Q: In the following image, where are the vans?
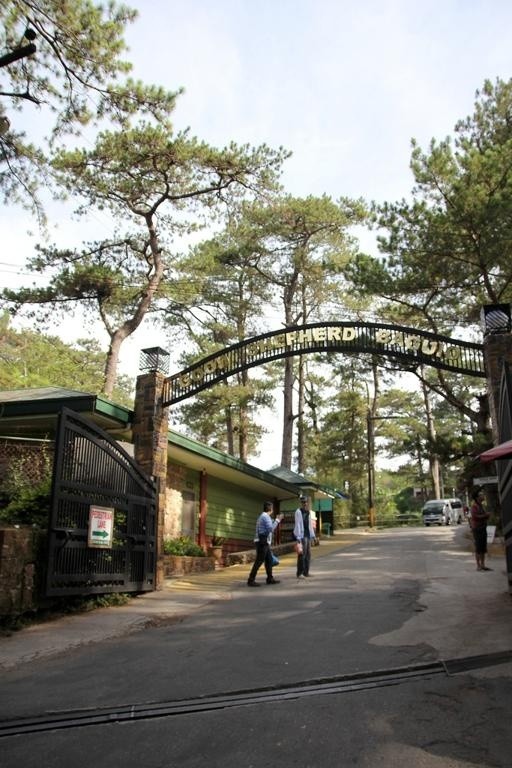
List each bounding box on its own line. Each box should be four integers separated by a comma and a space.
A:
422, 498, 464, 526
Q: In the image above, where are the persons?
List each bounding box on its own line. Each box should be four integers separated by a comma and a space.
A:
246, 502, 286, 587
292, 495, 318, 579
468, 489, 493, 572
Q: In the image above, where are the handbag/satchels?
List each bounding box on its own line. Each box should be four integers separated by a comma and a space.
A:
259, 534, 268, 543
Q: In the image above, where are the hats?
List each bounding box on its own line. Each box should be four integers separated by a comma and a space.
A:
298, 496, 307, 503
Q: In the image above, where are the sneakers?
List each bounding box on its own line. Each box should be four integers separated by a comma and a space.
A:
298, 574, 313, 580
266, 577, 281, 584
477, 567, 493, 572
247, 581, 261, 586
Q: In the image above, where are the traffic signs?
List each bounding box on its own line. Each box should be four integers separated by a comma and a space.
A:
87, 505, 115, 549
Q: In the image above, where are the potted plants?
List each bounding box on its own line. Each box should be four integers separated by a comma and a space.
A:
208, 534, 225, 559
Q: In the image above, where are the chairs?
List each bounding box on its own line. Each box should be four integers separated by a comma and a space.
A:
322, 523, 331, 536
